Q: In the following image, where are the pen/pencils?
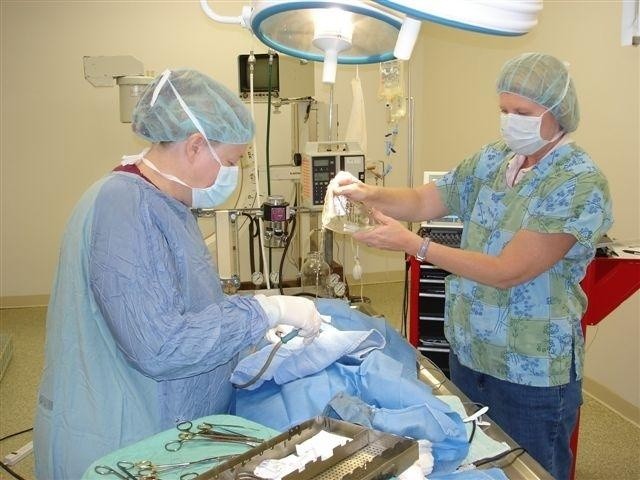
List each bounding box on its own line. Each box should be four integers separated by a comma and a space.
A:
623, 250, 640, 255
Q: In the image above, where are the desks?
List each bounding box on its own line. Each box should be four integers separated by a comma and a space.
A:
348, 298, 555, 480
82, 412, 283, 480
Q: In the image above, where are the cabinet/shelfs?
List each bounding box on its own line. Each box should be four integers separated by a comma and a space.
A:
405, 255, 454, 370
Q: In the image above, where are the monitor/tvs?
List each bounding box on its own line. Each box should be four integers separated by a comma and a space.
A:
237, 53, 315, 103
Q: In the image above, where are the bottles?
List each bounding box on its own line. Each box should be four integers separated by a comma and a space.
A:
296, 252, 330, 296
260, 194, 288, 249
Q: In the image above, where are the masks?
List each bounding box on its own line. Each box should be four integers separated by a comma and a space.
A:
182, 135, 240, 210
499, 99, 564, 158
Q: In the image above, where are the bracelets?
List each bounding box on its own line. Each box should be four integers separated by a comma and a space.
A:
413, 232, 433, 266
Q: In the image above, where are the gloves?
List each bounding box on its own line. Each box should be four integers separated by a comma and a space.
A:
254, 293, 321, 338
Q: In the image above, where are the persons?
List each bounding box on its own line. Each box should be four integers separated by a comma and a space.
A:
333, 52, 615, 478
30, 62, 333, 480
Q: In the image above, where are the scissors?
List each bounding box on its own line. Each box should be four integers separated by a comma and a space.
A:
95, 454, 264, 480
161, 419, 270, 455
